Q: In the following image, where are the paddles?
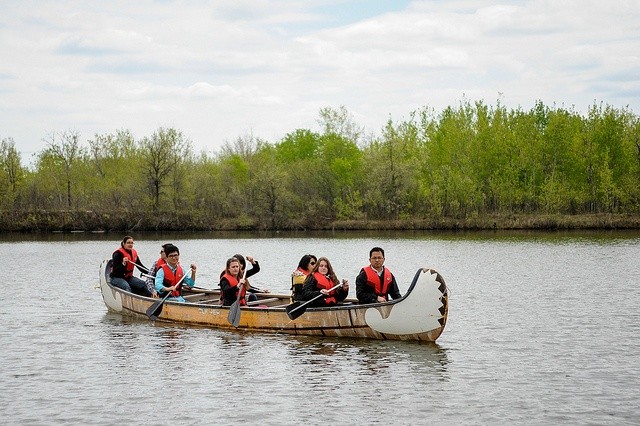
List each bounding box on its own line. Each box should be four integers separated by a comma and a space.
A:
285, 282, 343, 320
127, 259, 150, 272
140, 273, 220, 291
227, 259, 249, 328
145, 268, 192, 321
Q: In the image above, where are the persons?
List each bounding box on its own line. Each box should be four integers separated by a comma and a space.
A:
290, 255, 317, 304
355, 247, 402, 305
145, 244, 174, 299
109, 235, 152, 298
220, 256, 260, 308
219, 253, 271, 307
301, 257, 349, 307
155, 245, 196, 303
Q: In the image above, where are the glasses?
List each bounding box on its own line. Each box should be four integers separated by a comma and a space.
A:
371, 257, 384, 260
161, 250, 165, 253
309, 262, 315, 265
168, 254, 179, 257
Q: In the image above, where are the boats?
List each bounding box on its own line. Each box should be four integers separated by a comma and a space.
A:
99, 259, 449, 342
